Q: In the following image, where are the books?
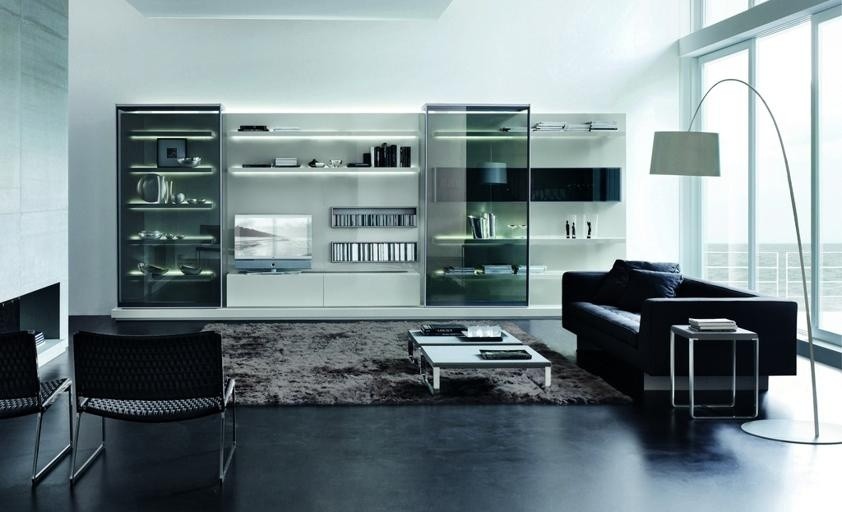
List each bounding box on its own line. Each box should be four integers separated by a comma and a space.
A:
237, 126, 300, 131
347, 142, 411, 167
420, 323, 469, 336
468, 212, 496, 239
688, 318, 738, 332
500, 120, 619, 133
479, 349, 532, 360
443, 263, 547, 276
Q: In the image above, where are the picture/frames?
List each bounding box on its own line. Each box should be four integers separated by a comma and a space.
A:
156, 137, 188, 168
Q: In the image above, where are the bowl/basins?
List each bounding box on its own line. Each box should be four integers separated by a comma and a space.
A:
176, 157, 201, 167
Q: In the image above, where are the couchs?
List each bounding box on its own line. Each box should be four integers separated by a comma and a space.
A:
559, 270, 798, 394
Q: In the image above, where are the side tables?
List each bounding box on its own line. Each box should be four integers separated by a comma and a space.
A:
668, 323, 760, 423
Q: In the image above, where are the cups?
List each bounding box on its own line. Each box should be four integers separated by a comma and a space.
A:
157, 176, 176, 205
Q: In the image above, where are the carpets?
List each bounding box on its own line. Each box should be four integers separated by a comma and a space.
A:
201, 320, 634, 408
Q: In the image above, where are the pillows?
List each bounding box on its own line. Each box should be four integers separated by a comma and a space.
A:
599, 258, 684, 314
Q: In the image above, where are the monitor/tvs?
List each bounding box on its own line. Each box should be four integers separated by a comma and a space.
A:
233, 212, 313, 273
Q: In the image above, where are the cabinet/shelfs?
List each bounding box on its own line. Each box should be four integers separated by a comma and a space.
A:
109, 102, 626, 323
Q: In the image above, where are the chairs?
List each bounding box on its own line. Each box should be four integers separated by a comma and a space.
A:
0, 328, 238, 489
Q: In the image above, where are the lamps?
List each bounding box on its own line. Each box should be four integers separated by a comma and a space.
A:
648, 76, 842, 447
